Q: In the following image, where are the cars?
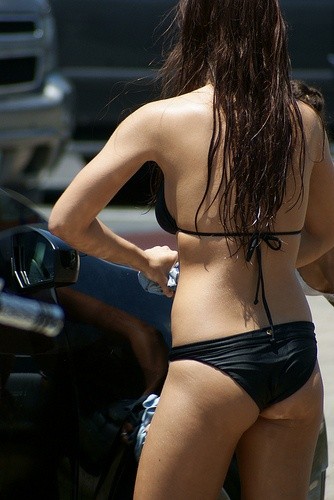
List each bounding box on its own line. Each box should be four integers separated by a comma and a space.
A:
1, 1, 333, 208
2, 188, 252, 499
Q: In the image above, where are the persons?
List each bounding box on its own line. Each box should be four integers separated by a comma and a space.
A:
47, 0, 332, 500
285, 80, 334, 306
